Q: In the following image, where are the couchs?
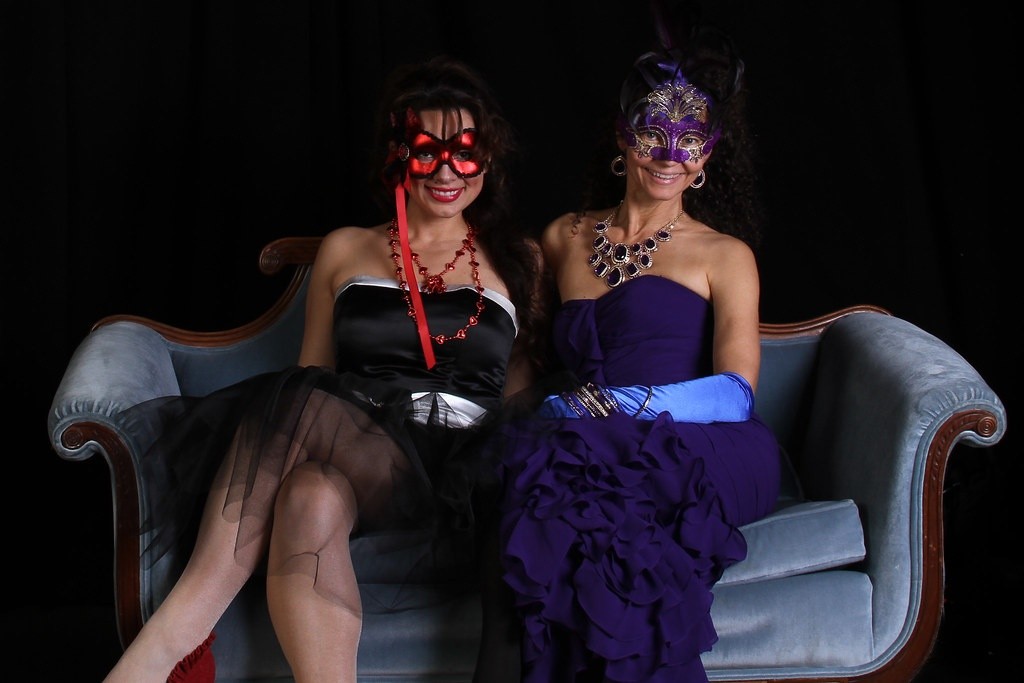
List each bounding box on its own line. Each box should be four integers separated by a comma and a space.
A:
46, 232, 1011, 683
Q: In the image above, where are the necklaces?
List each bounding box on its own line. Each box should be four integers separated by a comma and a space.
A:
386, 207, 486, 345
588, 198, 686, 292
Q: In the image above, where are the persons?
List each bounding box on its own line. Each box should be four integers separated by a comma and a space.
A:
91, 54, 540, 683
498, 51, 769, 682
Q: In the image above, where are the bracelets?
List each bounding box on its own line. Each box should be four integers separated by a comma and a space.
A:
559, 376, 654, 418
